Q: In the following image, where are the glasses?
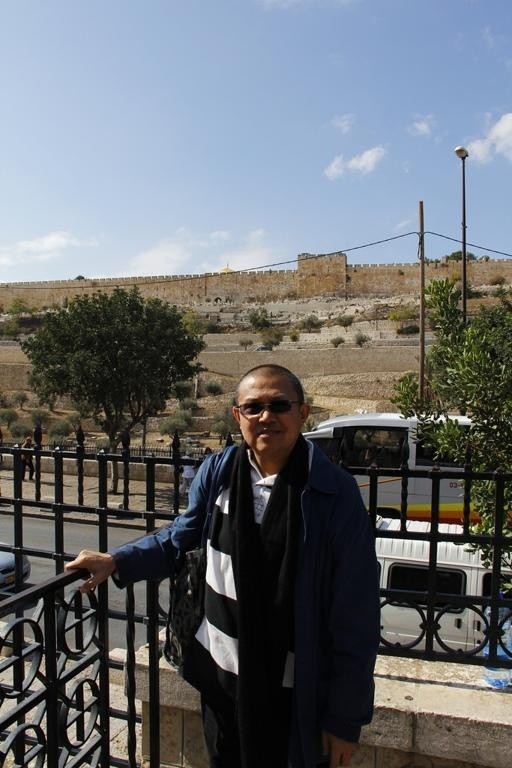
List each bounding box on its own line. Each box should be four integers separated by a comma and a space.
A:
236, 399, 301, 415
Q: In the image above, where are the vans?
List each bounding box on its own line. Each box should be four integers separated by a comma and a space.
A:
363, 515, 512, 656
301, 412, 512, 526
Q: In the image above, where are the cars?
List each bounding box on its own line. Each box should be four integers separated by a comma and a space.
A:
0, 540, 32, 592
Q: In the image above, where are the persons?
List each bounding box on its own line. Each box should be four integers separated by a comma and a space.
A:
64, 363, 381, 768
179, 448, 199, 496
202, 447, 212, 461
22, 436, 38, 482
187, 435, 192, 444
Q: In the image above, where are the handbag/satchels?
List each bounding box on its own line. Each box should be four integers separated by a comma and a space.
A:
163, 547, 207, 670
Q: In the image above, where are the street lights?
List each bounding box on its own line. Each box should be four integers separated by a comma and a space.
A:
449, 141, 471, 330
